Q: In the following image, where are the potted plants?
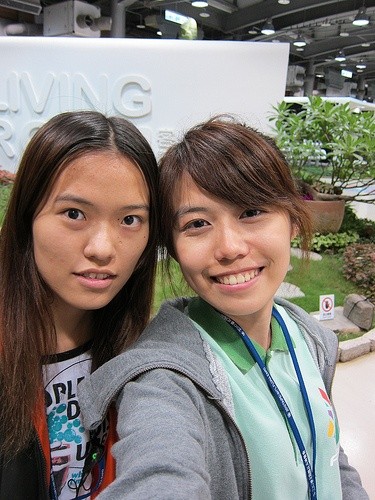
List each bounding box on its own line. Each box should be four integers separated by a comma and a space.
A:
269, 94, 375, 234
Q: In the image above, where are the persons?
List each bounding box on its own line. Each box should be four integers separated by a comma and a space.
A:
78, 115, 368, 500
0, 110, 159, 500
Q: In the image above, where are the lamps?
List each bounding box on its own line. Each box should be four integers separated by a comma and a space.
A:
261, 15, 275, 34
335, 48, 346, 62
352, 0, 371, 26
